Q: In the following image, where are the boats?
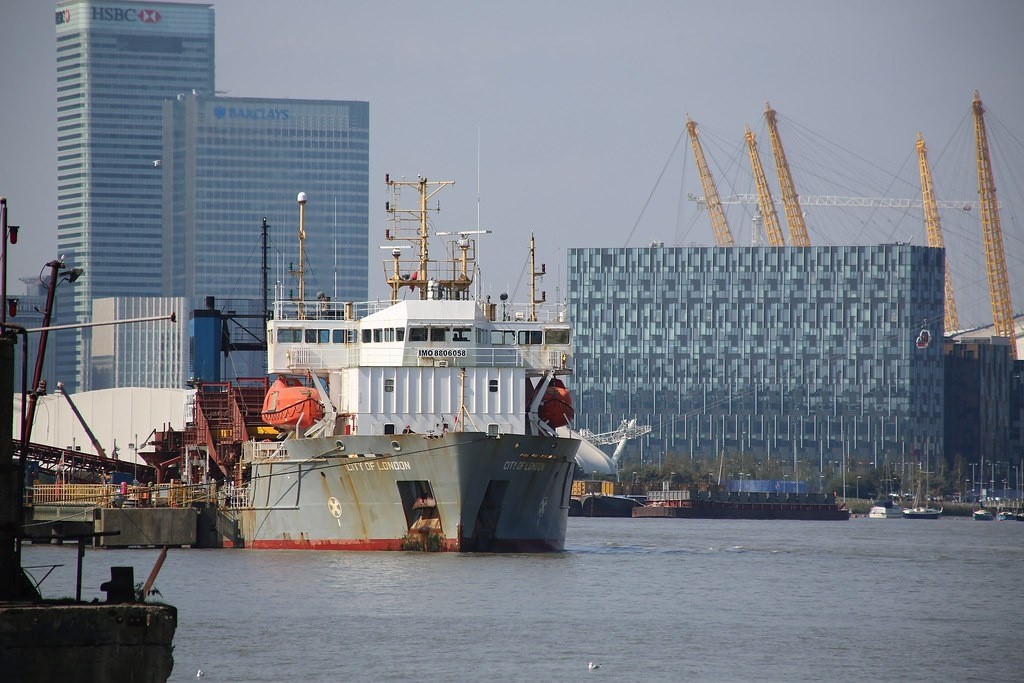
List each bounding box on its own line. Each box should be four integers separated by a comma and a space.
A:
996, 511, 1015, 521
1, 174, 853, 682
870, 506, 904, 520
1014, 513, 1024, 520
973, 510, 994, 520
903, 505, 945, 519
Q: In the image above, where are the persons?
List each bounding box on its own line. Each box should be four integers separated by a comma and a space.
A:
403, 425, 415, 434
